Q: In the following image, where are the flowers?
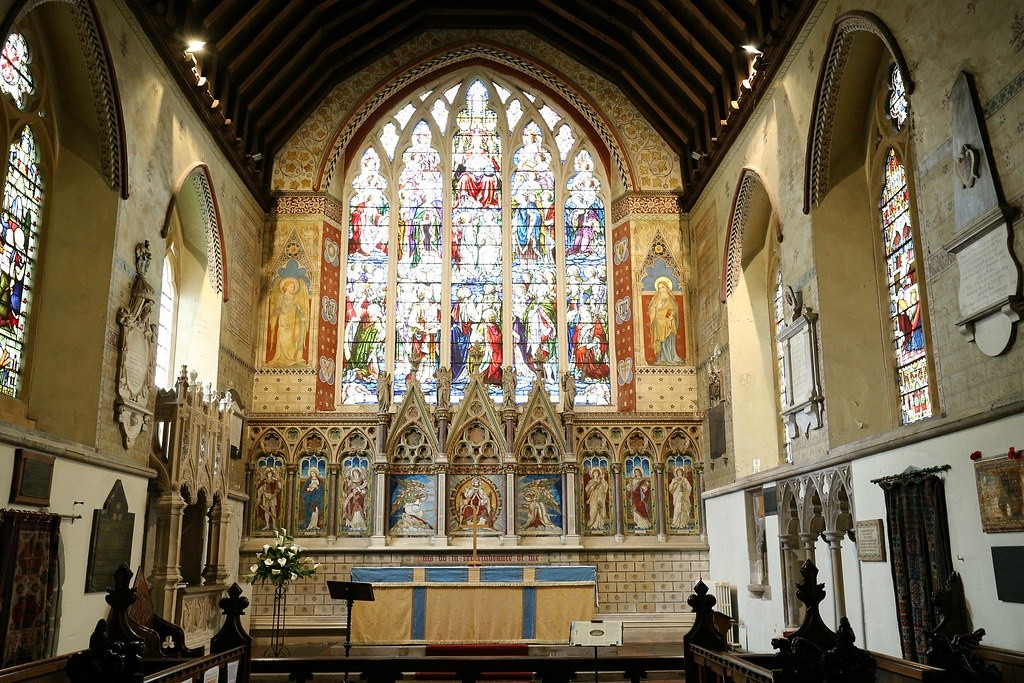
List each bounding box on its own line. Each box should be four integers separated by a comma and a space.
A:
242, 527, 320, 586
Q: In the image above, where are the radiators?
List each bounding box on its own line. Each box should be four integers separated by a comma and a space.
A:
694, 579, 736, 651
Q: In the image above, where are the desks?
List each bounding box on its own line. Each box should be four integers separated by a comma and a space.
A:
348, 565, 598, 645
